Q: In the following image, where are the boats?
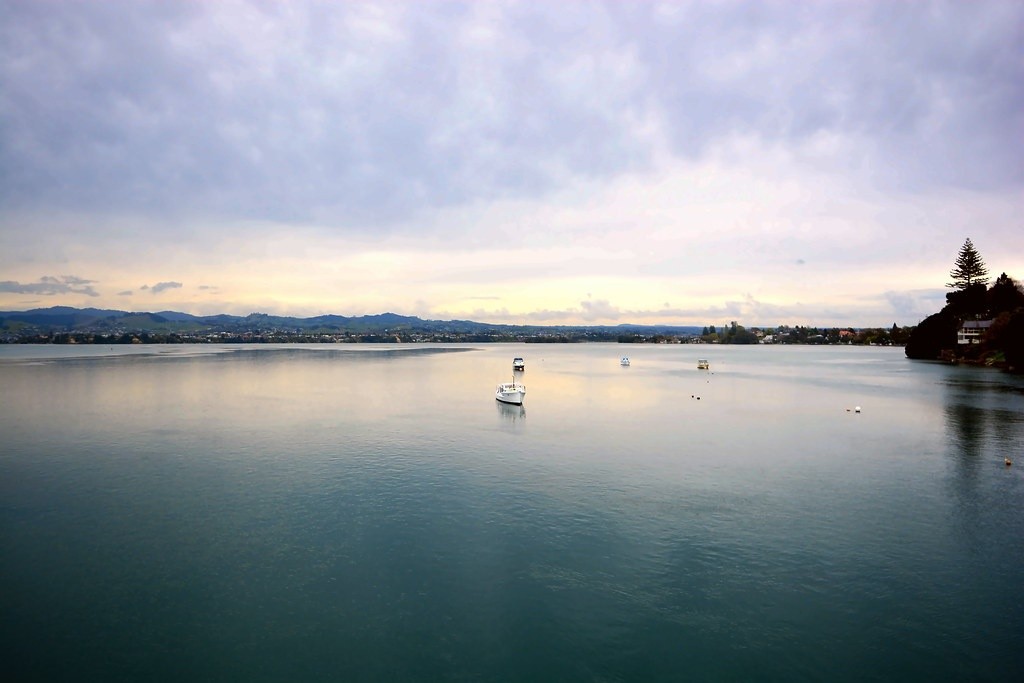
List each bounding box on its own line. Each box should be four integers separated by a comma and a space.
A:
495, 375, 525, 403
621, 357, 630, 365
512, 357, 524, 369
697, 359, 709, 369
